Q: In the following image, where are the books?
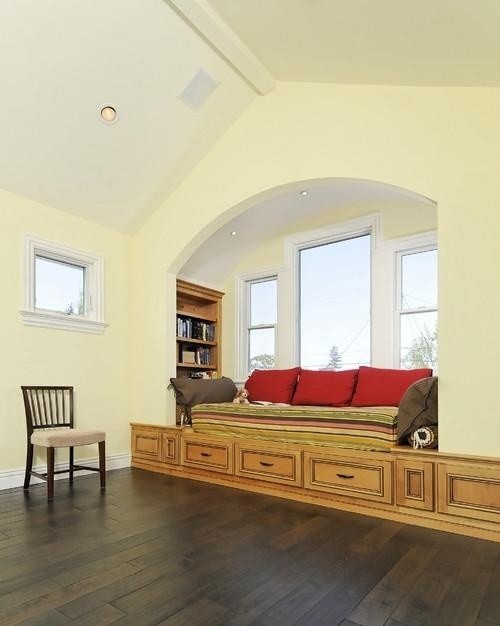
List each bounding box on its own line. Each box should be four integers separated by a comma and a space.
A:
176, 316, 217, 380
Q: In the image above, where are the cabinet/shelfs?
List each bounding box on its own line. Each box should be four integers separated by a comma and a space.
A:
130, 424, 500, 548
177, 281, 225, 379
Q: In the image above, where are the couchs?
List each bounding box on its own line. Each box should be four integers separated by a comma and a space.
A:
169, 365, 436, 451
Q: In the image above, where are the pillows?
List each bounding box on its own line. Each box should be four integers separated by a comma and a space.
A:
170, 367, 438, 439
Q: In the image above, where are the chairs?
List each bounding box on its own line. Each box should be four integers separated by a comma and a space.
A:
20, 387, 106, 500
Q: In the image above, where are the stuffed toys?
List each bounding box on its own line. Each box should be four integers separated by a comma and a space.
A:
232, 389, 251, 404
